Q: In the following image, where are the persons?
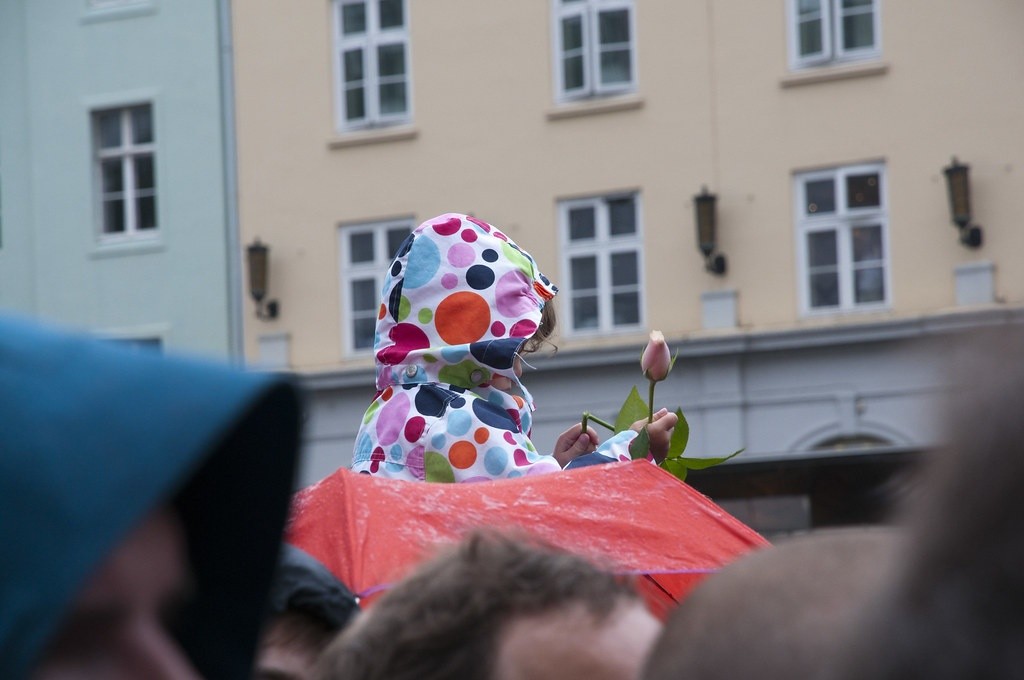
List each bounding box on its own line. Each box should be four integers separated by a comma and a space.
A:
246, 536, 365, 680
344, 212, 682, 479
0, 302, 314, 680
303, 525, 668, 680
866, 339, 1023, 678
641, 518, 927, 680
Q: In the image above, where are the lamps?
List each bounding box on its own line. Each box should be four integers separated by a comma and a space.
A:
247, 238, 278, 320
943, 157, 981, 246
693, 187, 725, 275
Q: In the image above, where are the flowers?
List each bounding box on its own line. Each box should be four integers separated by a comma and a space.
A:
581, 329, 745, 484
